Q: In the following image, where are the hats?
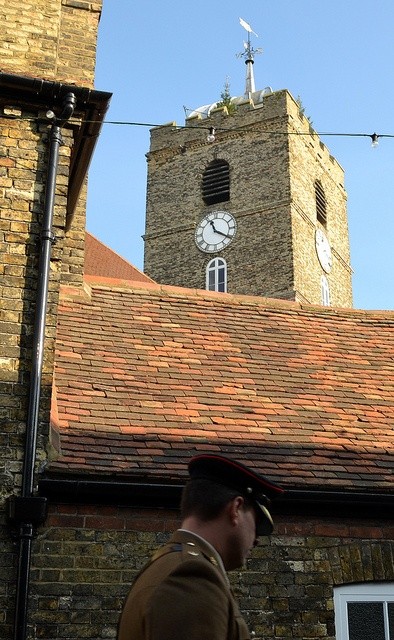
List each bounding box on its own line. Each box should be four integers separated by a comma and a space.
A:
188, 454, 284, 535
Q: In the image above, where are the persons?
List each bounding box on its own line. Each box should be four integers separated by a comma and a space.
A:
116, 454, 284, 640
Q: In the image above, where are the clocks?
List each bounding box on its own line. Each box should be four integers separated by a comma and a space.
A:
315, 229, 333, 274
193, 209, 238, 255
